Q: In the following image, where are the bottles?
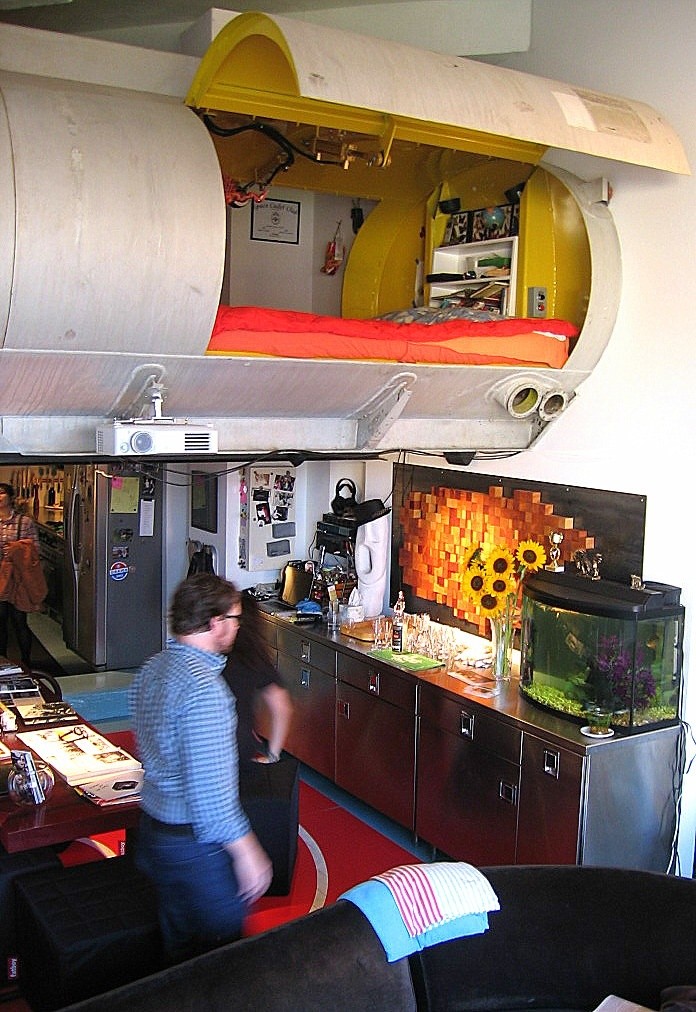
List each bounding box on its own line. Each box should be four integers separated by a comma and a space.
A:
391, 598, 408, 654
8, 760, 54, 808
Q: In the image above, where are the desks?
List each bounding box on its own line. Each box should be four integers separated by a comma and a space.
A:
0, 652, 146, 865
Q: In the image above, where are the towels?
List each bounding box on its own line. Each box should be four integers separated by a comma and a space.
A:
336, 861, 502, 964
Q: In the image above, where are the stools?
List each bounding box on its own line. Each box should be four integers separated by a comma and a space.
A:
11, 854, 206, 1012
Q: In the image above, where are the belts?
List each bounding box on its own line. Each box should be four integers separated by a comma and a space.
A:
138, 811, 198, 836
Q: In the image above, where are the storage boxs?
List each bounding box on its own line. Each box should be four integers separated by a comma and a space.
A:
519, 584, 686, 733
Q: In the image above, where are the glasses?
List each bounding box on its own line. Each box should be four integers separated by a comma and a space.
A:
0, 490, 7, 494
224, 613, 245, 624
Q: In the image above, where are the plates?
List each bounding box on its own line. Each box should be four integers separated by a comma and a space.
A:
580, 726, 614, 738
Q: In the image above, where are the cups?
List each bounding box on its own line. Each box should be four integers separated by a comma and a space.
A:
345, 617, 355, 629
371, 612, 455, 672
327, 601, 342, 631
587, 706, 613, 735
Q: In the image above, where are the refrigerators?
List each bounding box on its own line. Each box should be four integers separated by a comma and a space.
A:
61, 463, 161, 669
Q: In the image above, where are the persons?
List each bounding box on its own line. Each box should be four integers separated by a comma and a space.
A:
221, 591, 302, 898
128, 571, 274, 966
0, 481, 48, 670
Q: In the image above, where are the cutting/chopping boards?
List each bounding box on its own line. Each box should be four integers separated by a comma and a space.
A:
339, 616, 393, 642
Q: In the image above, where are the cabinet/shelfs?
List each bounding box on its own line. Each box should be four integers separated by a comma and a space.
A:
247, 616, 585, 872
426, 237, 518, 316
